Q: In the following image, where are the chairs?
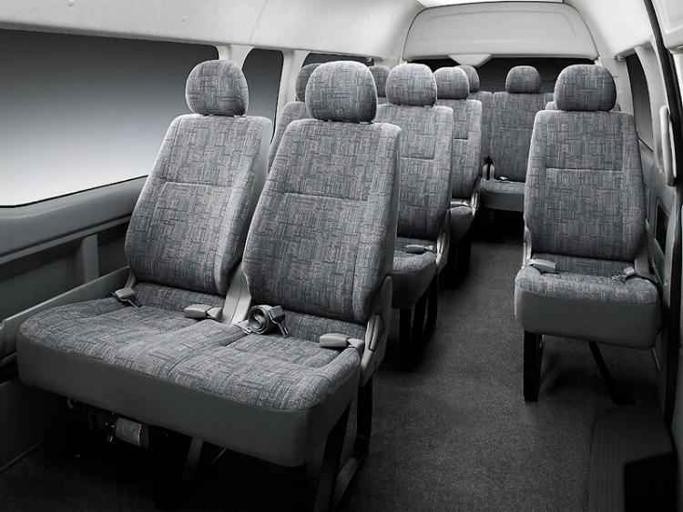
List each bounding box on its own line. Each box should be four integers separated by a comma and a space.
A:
510, 63, 662, 407
266, 59, 551, 374
14, 59, 405, 507
